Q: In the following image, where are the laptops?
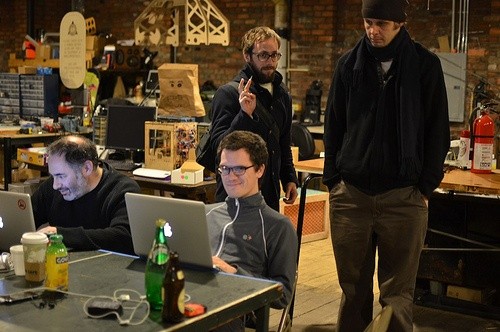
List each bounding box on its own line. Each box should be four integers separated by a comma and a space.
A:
125, 193, 220, 272
0, 191, 36, 252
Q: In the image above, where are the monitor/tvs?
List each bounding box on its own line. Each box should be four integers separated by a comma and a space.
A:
145, 70, 160, 93
105, 105, 156, 163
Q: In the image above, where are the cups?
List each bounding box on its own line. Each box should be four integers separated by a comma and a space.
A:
134, 88, 142, 97
21, 232, 49, 281
10, 245, 25, 275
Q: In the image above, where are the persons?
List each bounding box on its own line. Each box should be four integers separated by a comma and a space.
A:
28, 135, 143, 256
196, 28, 299, 214
202, 132, 297, 332
321, 0, 451, 332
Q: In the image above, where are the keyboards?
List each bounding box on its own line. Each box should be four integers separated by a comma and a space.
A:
104, 160, 135, 171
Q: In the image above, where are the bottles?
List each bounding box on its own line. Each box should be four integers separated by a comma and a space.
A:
145, 226, 170, 310
492, 154, 496, 170
46, 235, 68, 291
451, 141, 460, 159
458, 130, 470, 167
145, 96, 156, 107
321, 112, 324, 123
164, 251, 186, 326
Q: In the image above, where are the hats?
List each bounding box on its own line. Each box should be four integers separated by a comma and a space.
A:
361, 0, 406, 22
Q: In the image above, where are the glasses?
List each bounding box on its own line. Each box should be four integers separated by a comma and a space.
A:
216, 165, 255, 176
250, 51, 281, 62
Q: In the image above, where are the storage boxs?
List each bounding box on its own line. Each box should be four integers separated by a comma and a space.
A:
16, 148, 48, 166
0, 73, 60, 121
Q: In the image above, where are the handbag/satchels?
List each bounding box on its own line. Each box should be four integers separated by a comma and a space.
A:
195, 81, 252, 174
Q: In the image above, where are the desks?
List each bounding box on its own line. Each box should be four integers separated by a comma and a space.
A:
0, 130, 220, 205
284, 157, 500, 327
0, 248, 285, 332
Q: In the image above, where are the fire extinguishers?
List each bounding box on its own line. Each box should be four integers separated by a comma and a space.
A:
470, 102, 500, 174
